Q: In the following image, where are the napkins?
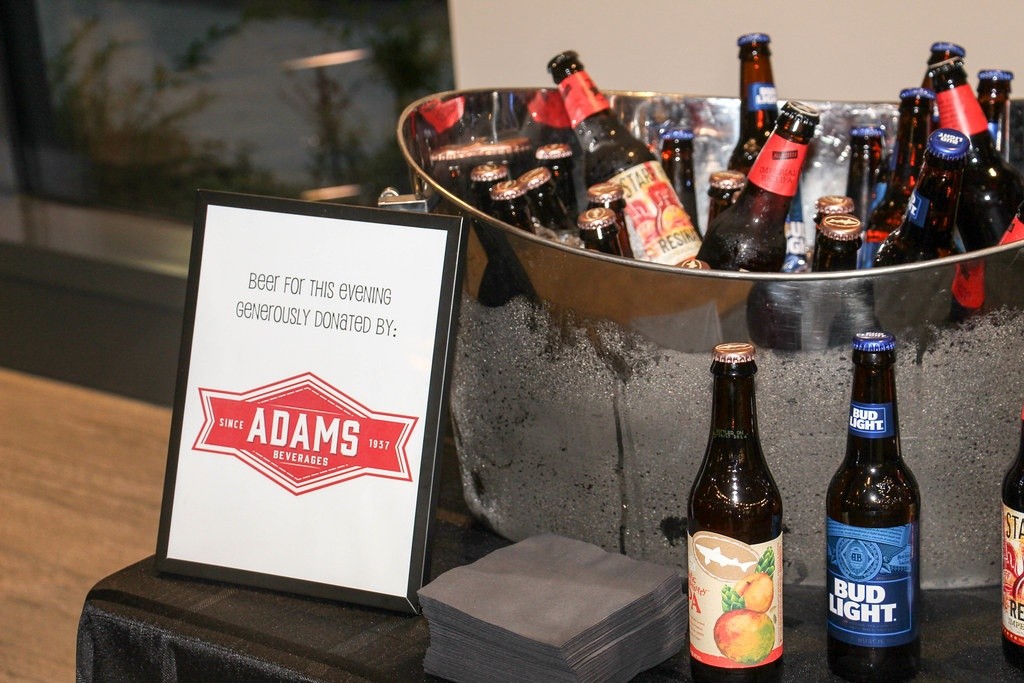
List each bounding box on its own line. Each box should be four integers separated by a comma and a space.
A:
416, 535, 692, 683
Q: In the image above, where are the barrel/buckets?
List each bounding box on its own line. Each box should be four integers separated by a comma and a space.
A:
394, 84, 1024, 591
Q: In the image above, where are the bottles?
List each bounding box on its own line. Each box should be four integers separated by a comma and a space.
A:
999, 403, 1024, 662
824, 330, 923, 683
467, 143, 636, 267
811, 121, 884, 274
693, 28, 821, 274
685, 340, 785, 683
546, 46, 702, 267
864, 39, 1024, 269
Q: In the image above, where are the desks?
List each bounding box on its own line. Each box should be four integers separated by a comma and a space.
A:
76, 511, 1024, 683
